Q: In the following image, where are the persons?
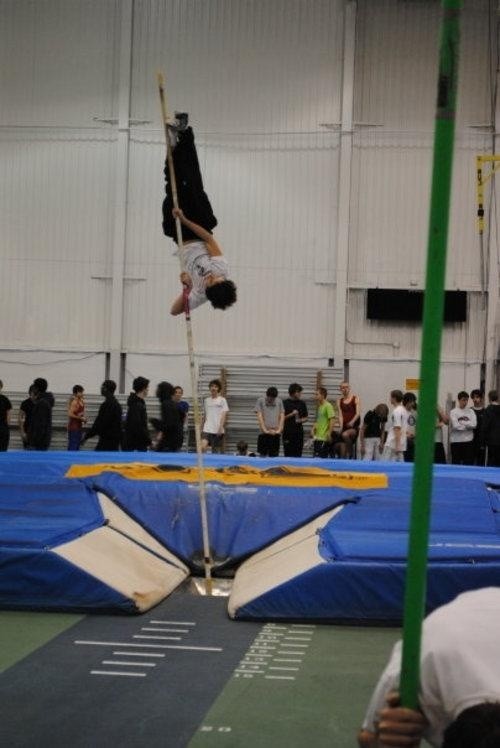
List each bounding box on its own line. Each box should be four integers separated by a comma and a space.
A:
68, 385, 86, 451
381, 390, 406, 462
359, 586, 500, 748
338, 382, 360, 458
436, 404, 449, 464
449, 392, 476, 466
360, 404, 389, 463
163, 111, 237, 315
402, 392, 417, 462
149, 382, 183, 452
81, 380, 122, 451
19, 378, 54, 446
234, 441, 255, 457
255, 387, 284, 457
0, 380, 11, 451
470, 390, 487, 466
125, 377, 157, 451
26, 385, 52, 450
203, 380, 229, 453
311, 388, 335, 458
485, 391, 500, 467
174, 387, 189, 431
282, 384, 307, 457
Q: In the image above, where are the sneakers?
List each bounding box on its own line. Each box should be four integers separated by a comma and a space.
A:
176, 109, 187, 130
164, 121, 176, 139
